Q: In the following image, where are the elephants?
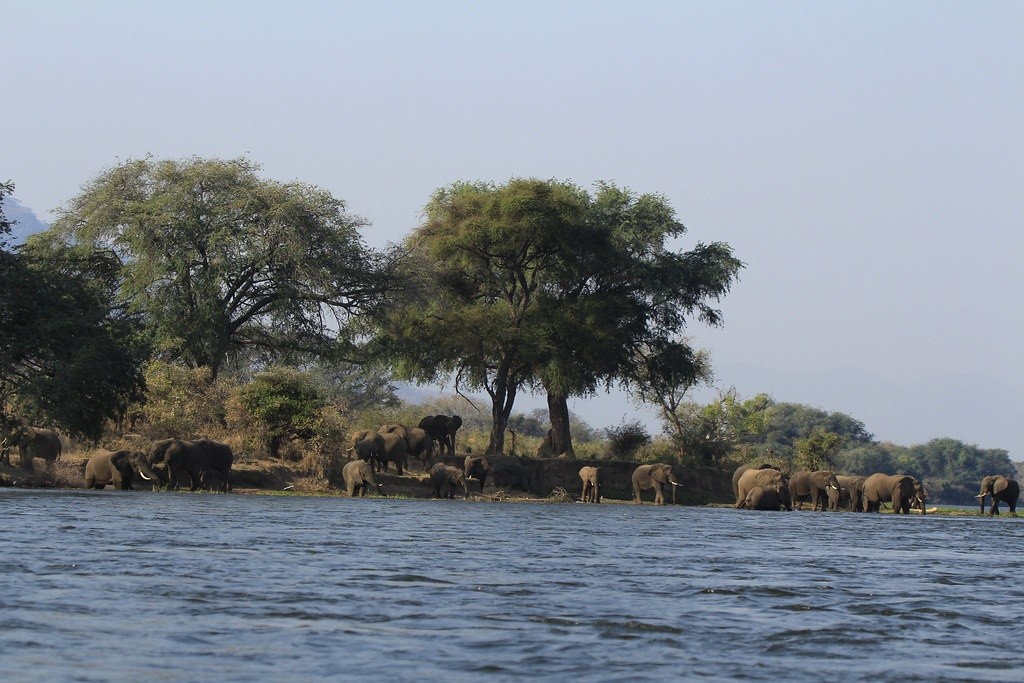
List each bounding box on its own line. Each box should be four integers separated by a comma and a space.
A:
0, 414, 1020, 518
